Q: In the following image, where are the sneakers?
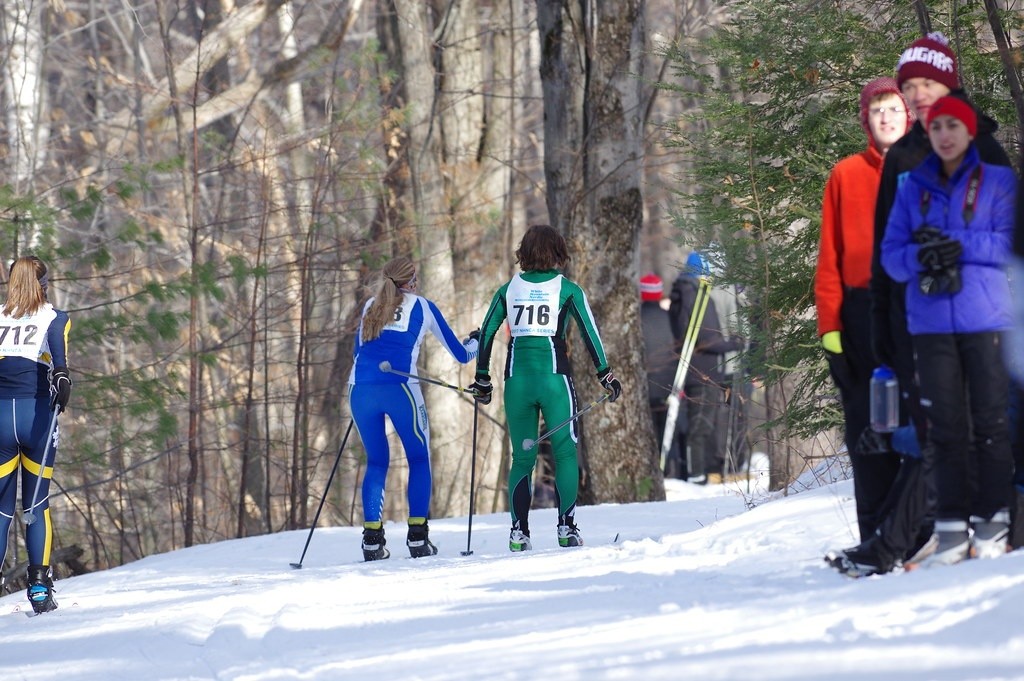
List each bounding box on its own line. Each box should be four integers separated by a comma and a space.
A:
556, 523, 585, 547
509, 528, 533, 552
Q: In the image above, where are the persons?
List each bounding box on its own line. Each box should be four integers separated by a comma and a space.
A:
470, 223, 623, 554
637, 252, 750, 486
868, 33, 1021, 526
813, 78, 933, 575
878, 89, 1024, 535
0, 256, 72, 613
346, 255, 479, 562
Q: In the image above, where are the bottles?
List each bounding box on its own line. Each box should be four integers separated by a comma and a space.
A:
868, 360, 899, 433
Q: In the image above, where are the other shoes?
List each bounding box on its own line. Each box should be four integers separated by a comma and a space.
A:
688, 473, 723, 484
822, 518, 938, 580
966, 505, 1012, 560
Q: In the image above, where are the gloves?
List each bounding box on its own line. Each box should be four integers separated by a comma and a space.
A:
871, 325, 891, 369
731, 331, 744, 349
823, 330, 849, 371
50, 368, 72, 413
469, 328, 480, 340
913, 226, 942, 243
916, 239, 964, 272
597, 366, 622, 402
472, 374, 493, 405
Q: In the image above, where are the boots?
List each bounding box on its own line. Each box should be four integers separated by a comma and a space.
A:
26, 565, 58, 614
361, 520, 391, 562
406, 518, 438, 558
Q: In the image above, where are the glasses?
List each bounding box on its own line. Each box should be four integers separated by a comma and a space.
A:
870, 104, 905, 116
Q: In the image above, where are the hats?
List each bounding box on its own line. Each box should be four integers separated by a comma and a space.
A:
897, 31, 958, 90
684, 253, 711, 278
861, 76, 913, 145
924, 88, 977, 137
639, 274, 664, 302
